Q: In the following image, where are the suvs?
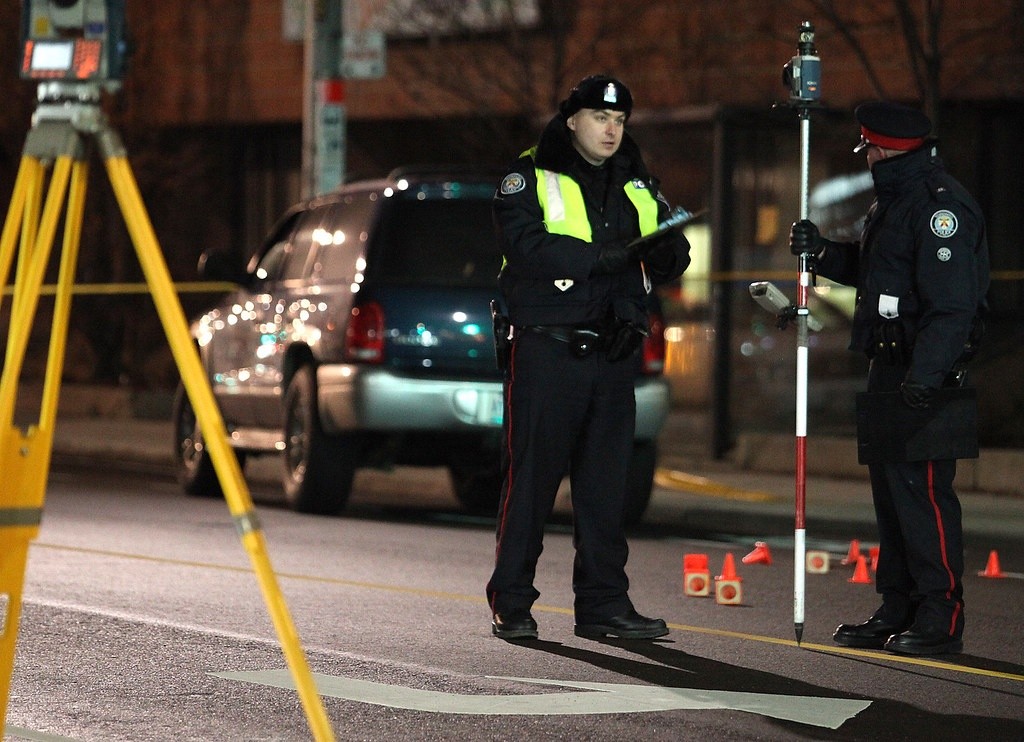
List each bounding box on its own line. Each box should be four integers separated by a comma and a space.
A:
171, 175, 670, 538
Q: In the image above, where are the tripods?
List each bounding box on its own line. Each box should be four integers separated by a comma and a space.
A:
0, 78, 339, 742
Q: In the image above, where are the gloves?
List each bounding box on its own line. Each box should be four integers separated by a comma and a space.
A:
594, 237, 646, 275
651, 225, 691, 275
788, 220, 822, 260
900, 379, 935, 410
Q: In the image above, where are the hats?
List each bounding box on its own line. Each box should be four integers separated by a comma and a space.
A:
568, 74, 631, 122
853, 102, 933, 155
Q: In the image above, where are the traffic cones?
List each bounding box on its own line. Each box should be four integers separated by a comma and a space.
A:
805, 550, 830, 575
682, 553, 711, 597
715, 580, 743, 607
840, 539, 861, 565
847, 556, 871, 584
869, 547, 881, 573
714, 550, 742, 583
744, 542, 772, 566
978, 550, 1009, 580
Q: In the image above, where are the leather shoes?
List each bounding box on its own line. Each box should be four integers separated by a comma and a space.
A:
574, 591, 669, 639
832, 604, 910, 649
491, 606, 538, 640
883, 624, 964, 655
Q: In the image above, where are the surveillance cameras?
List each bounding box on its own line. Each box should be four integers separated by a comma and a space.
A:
747, 282, 792, 315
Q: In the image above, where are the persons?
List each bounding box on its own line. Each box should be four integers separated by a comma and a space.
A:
486, 73, 692, 639
788, 96, 988, 657
